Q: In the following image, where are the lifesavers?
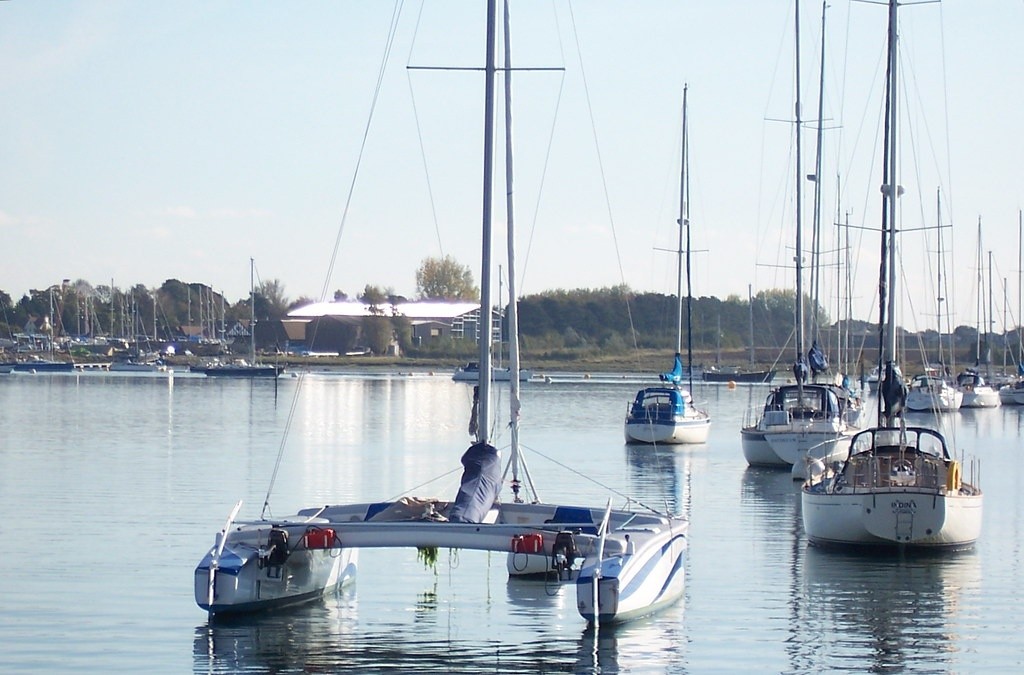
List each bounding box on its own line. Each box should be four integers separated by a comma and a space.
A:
946, 461, 962, 492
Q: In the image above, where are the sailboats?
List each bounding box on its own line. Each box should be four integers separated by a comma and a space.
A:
451, 264, 534, 382
739, 0, 1023, 553
194, 0, 691, 628
188, 257, 289, 378
701, 283, 779, 383
624, 82, 713, 446
0, 275, 225, 379
